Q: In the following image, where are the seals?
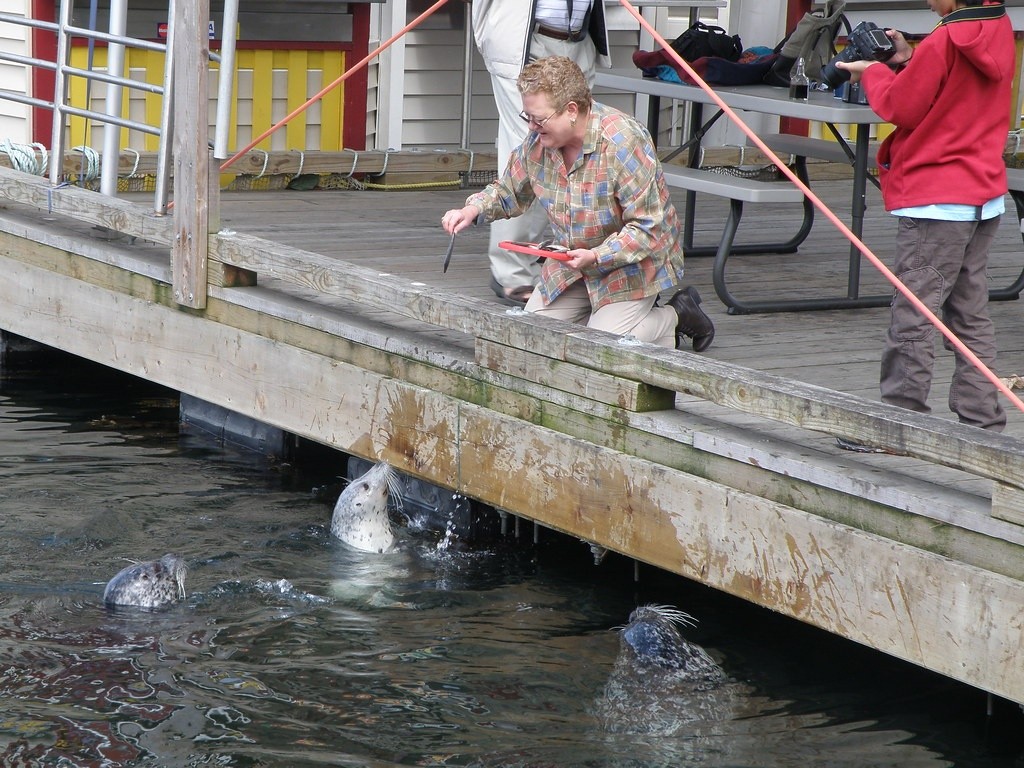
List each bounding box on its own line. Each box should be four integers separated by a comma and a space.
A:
104, 553, 189, 610
593, 605, 743, 766
328, 459, 411, 606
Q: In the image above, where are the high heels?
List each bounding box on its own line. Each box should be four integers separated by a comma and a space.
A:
665, 287, 714, 351
651, 293, 682, 350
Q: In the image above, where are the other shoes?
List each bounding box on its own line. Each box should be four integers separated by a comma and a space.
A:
836, 436, 895, 452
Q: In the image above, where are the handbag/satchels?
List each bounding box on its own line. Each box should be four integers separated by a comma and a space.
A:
634, 1, 854, 91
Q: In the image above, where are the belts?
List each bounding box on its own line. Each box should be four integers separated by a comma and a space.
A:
531, 22, 589, 41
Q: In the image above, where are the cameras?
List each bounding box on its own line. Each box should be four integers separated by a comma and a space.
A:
820, 21, 897, 90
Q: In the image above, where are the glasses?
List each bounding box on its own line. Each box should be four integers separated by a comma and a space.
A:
519, 99, 577, 131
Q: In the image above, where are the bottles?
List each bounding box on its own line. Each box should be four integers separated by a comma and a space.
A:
788, 57, 809, 103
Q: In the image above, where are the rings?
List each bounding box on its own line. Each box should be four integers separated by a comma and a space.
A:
441, 217, 444, 222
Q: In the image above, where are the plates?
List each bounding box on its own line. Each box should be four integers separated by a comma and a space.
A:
498, 240, 575, 261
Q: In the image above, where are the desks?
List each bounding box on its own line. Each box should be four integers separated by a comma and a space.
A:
588, 63, 1024, 311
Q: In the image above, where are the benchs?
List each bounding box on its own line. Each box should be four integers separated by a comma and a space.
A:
656, 131, 1023, 311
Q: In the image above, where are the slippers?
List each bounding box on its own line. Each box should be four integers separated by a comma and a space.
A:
490, 279, 536, 307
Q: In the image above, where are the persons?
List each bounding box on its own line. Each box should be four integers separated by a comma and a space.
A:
835, 0, 1016, 452
442, 56, 715, 353
471, 0, 612, 307
